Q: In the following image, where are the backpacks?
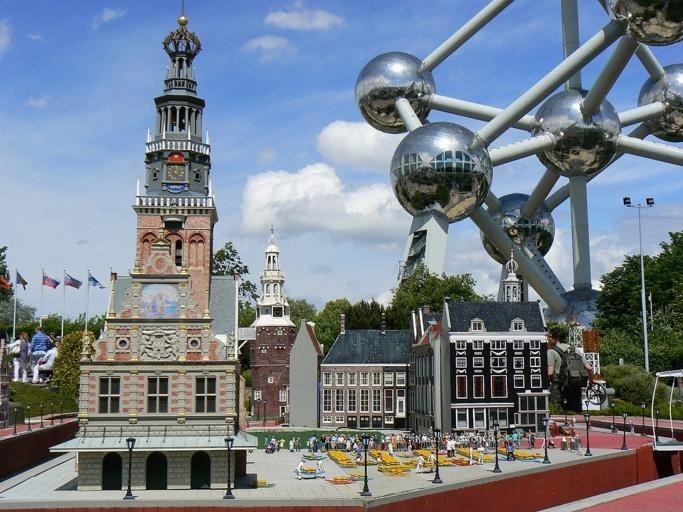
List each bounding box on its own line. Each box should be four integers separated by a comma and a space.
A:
551, 345, 589, 389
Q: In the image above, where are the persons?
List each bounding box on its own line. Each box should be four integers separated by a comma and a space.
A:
263, 417, 582, 480
546, 326, 593, 413
5, 326, 62, 384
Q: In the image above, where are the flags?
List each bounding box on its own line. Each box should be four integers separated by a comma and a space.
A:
0, 274, 10, 289
41, 270, 60, 290
63, 271, 82, 289
87, 271, 107, 290
16, 271, 28, 291
